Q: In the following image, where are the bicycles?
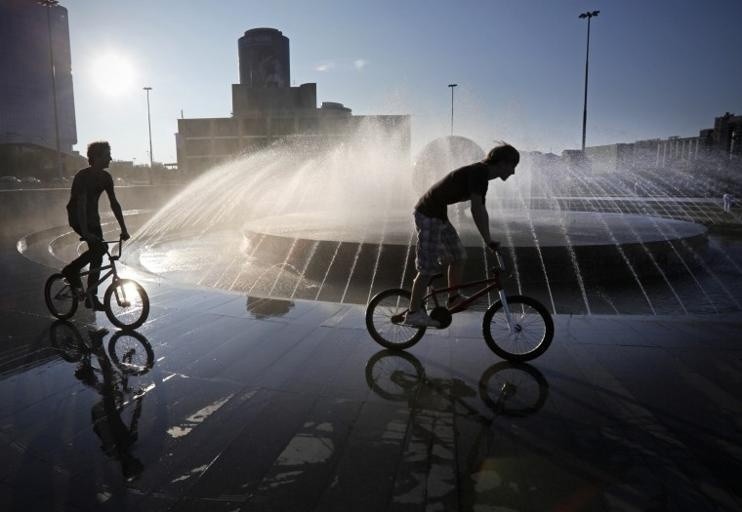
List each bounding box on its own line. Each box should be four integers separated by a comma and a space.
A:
44, 233, 149, 330
366, 245, 554, 362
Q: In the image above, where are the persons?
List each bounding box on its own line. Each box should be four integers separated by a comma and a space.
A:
402, 143, 520, 327
60, 139, 131, 312
722, 188, 735, 214
72, 326, 147, 486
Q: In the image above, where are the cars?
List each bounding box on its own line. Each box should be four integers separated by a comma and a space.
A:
0, 176, 75, 188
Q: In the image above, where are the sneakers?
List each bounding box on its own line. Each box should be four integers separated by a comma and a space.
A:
446, 294, 470, 307
85, 296, 104, 310
61, 265, 83, 287
405, 308, 440, 327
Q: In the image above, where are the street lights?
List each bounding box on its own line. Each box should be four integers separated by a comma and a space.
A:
578, 10, 599, 175
143, 87, 153, 185
449, 84, 458, 137
